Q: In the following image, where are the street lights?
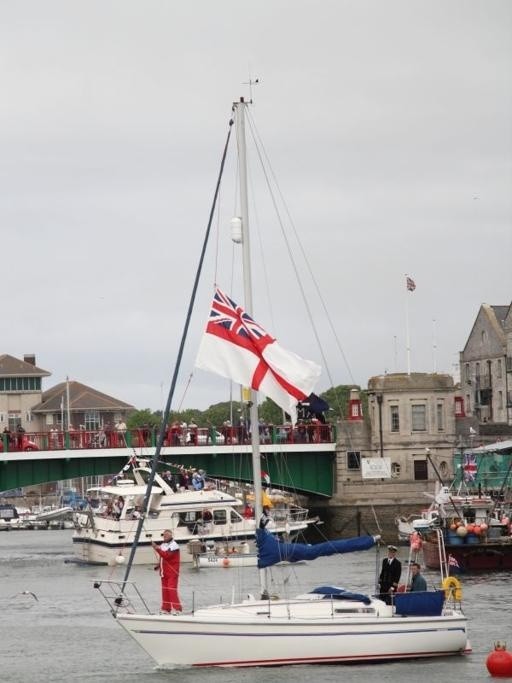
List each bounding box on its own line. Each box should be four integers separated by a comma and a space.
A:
374, 391, 386, 456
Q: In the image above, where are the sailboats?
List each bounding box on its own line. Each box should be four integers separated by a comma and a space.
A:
90, 73, 476, 674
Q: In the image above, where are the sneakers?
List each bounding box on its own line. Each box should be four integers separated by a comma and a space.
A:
157, 610, 172, 616
172, 610, 184, 616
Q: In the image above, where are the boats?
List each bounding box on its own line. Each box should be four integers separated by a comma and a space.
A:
413, 428, 511, 577
66, 450, 326, 567
0, 496, 79, 531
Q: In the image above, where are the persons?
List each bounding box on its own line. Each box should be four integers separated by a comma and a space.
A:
258, 418, 266, 435
50, 425, 59, 448
96, 419, 126, 447
410, 563, 427, 591
284, 413, 318, 443
3, 425, 25, 449
170, 419, 197, 444
375, 544, 401, 605
98, 452, 272, 523
224, 415, 246, 445
152, 531, 186, 615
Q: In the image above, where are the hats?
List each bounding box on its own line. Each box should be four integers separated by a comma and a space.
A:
387, 544, 398, 553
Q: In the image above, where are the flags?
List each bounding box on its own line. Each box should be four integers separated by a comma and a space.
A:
197, 289, 318, 417
460, 453, 478, 484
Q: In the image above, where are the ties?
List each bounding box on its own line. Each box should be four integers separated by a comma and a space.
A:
388, 560, 391, 565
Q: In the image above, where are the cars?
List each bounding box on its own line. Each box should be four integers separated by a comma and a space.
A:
184, 423, 289, 445
1, 436, 38, 451
